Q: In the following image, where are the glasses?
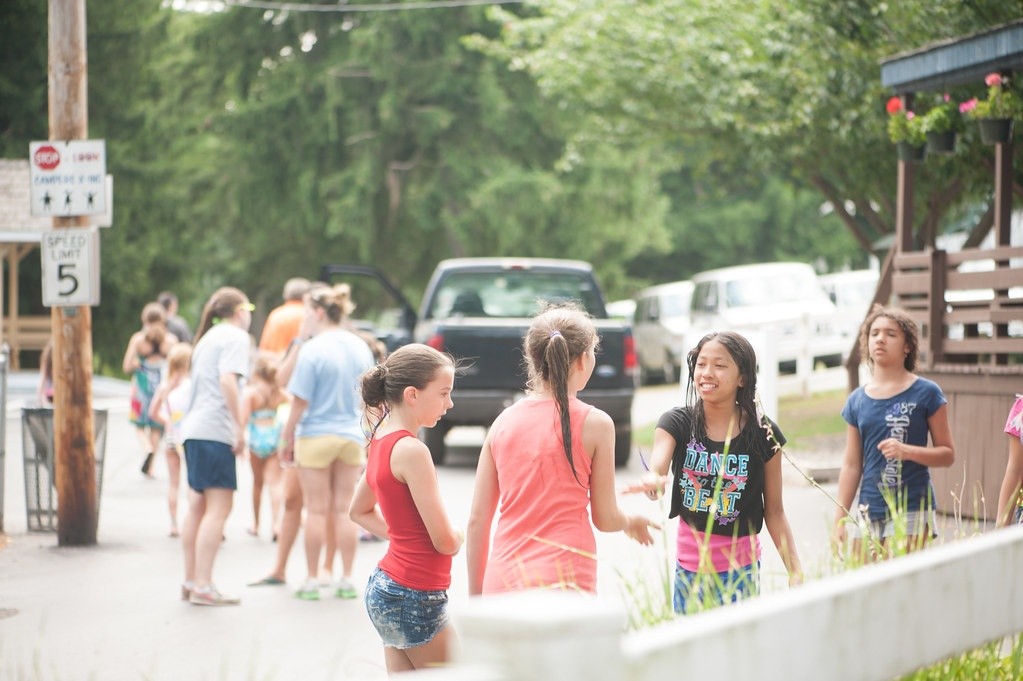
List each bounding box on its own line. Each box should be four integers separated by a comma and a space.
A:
238, 303, 255, 312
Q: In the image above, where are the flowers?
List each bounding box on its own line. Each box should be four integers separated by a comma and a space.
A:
887, 73, 1023, 148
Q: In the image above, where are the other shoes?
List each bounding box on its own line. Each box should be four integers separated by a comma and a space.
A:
179, 583, 192, 599
295, 578, 320, 599
337, 582, 356, 598
190, 587, 237, 605
249, 576, 283, 586
141, 452, 155, 473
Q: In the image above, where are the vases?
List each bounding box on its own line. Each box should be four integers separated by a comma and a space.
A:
925, 130, 956, 151
896, 142, 924, 161
979, 118, 1014, 146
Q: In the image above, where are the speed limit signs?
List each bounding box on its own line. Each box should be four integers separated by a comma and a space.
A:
41, 225, 101, 310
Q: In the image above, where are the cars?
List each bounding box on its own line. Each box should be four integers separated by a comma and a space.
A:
607, 259, 891, 390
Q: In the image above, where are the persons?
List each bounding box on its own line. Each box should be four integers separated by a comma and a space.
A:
158, 292, 191, 342
35, 340, 53, 404
347, 344, 464, 675
123, 303, 179, 471
148, 343, 191, 535
242, 279, 388, 587
622, 332, 804, 615
177, 288, 255, 606
279, 284, 376, 599
996, 397, 1023, 529
831, 312, 956, 562
467, 309, 662, 594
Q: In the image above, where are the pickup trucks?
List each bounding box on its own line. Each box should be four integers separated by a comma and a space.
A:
315, 255, 639, 468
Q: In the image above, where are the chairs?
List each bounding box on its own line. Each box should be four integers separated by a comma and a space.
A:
451, 294, 484, 318
